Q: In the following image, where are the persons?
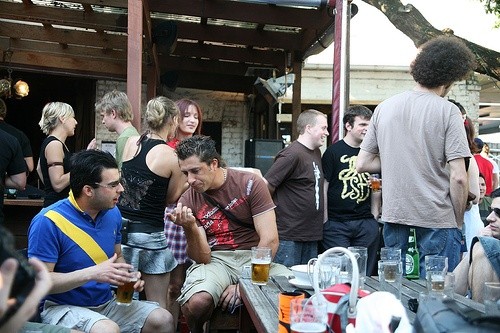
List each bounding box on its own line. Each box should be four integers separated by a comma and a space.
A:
96, 90, 142, 178
119, 96, 191, 311
0, 102, 98, 227
0, 256, 52, 333
264, 108, 329, 269
318, 103, 382, 279
355, 33, 478, 281
163, 98, 198, 332
446, 98, 500, 306
166, 134, 295, 333
27, 150, 174, 333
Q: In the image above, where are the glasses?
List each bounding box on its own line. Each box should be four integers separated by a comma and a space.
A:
94, 171, 122, 191
488, 206, 500, 218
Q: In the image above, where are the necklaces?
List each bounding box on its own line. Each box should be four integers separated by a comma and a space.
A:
218, 167, 227, 180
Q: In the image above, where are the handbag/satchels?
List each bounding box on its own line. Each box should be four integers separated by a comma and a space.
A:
302, 247, 371, 333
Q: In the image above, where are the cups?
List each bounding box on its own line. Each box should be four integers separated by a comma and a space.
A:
377, 247, 403, 301
290, 298, 329, 333
425, 255, 455, 298
345, 246, 367, 290
483, 282, 500, 313
307, 254, 341, 291
251, 247, 272, 286
116, 277, 137, 307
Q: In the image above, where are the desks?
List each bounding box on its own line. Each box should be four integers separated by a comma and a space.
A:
3, 195, 47, 259
239, 274, 500, 333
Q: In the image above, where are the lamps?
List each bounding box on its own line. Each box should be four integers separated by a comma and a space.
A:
0, 50, 30, 100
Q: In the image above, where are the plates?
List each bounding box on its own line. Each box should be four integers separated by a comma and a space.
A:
289, 279, 314, 289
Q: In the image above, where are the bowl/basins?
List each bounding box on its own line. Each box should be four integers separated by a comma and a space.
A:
291, 265, 335, 283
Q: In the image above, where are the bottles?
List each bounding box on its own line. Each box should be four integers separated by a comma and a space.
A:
405, 228, 420, 280
372, 174, 380, 191
